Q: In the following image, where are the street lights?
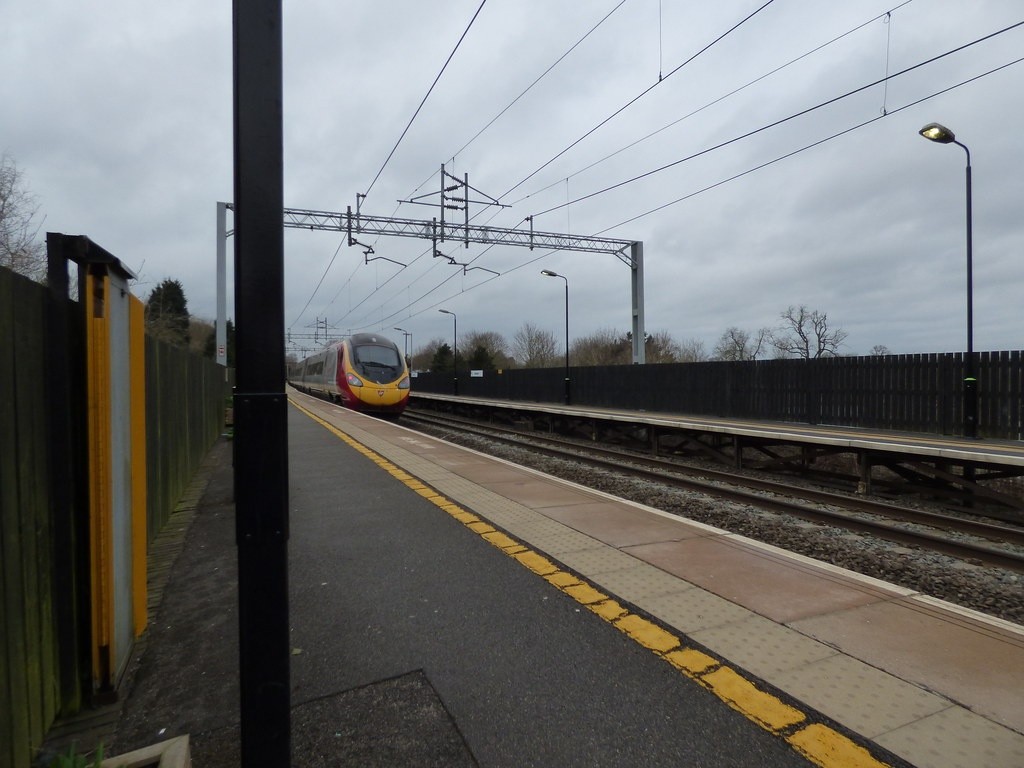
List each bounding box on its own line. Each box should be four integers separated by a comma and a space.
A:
541, 269, 570, 405
921, 122, 977, 439
394, 327, 407, 364
439, 309, 457, 396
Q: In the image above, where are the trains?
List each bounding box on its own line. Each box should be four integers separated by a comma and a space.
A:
286, 333, 409, 418
